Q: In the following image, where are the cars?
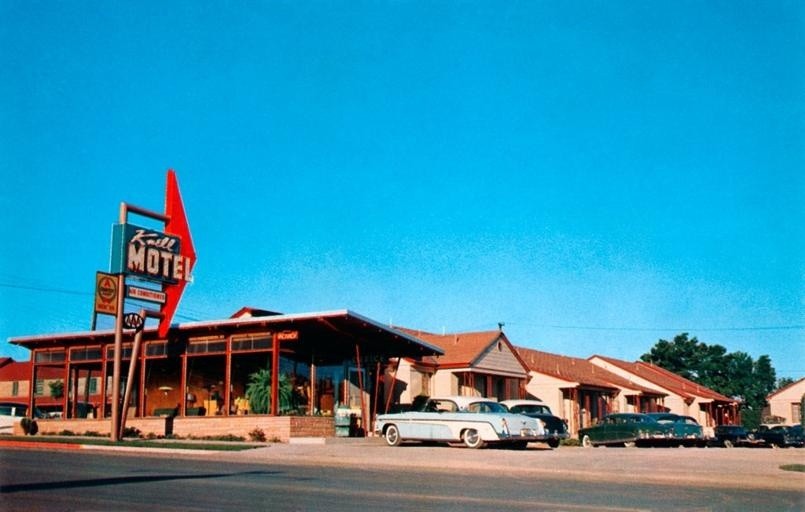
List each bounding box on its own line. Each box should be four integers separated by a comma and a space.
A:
376, 395, 569, 450
579, 410, 701, 447
716, 425, 805, 448
